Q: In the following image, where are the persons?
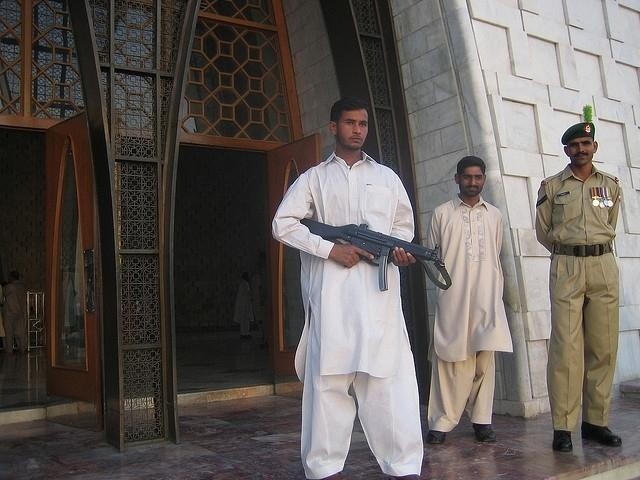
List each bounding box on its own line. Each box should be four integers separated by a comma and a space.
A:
536, 122, 622, 452
272, 98, 425, 479
235, 271, 254, 337
426, 156, 513, 445
0, 271, 26, 353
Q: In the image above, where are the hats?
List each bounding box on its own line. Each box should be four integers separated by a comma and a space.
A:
561, 122, 595, 145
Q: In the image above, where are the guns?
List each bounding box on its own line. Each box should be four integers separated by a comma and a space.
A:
300, 218, 452, 291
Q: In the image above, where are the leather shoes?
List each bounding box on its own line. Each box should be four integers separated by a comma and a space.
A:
581, 422, 622, 447
474, 424, 496, 441
552, 431, 573, 452
427, 431, 445, 444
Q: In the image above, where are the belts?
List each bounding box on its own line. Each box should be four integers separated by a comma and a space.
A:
553, 244, 613, 257
